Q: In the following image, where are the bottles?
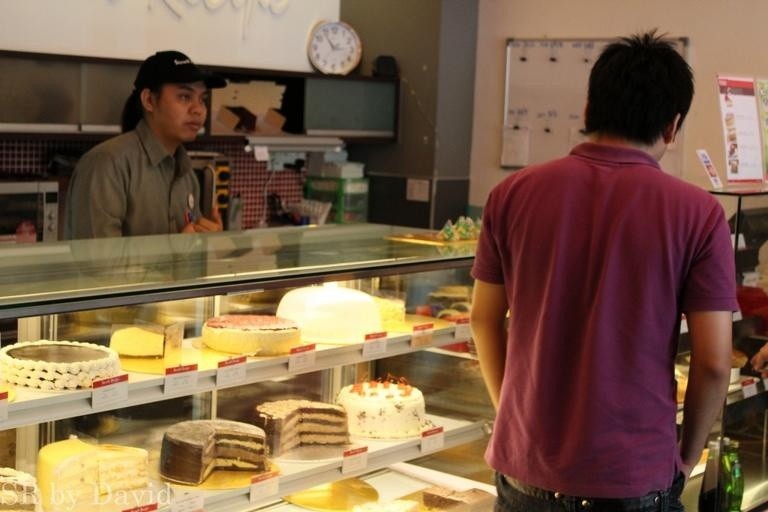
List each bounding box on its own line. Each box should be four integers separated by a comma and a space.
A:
698, 437, 743, 512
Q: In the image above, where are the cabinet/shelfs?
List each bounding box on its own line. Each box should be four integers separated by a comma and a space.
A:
675, 189, 767, 512
2, 222, 500, 508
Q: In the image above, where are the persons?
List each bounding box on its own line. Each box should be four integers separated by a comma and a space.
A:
469, 27, 744, 512
59, 49, 226, 327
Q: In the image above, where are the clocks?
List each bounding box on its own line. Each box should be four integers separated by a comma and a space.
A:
307, 19, 363, 77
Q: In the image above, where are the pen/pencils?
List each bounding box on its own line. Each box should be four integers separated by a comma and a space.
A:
183, 210, 195, 223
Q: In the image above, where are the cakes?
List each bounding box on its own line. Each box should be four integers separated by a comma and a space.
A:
156, 418, 267, 485
0, 339, 120, 391
111, 325, 184, 375
0, 466, 39, 511
337, 380, 426, 439
39, 439, 154, 512
255, 399, 350, 459
202, 314, 299, 357
277, 287, 381, 344
372, 296, 406, 332
423, 486, 497, 512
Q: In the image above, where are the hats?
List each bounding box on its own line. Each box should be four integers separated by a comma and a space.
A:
136, 50, 226, 88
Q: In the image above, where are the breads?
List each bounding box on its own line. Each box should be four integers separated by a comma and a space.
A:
413, 287, 472, 318
675, 366, 689, 403
685, 347, 748, 368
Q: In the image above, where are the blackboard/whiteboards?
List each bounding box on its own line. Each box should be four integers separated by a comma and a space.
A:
499, 37, 690, 170
